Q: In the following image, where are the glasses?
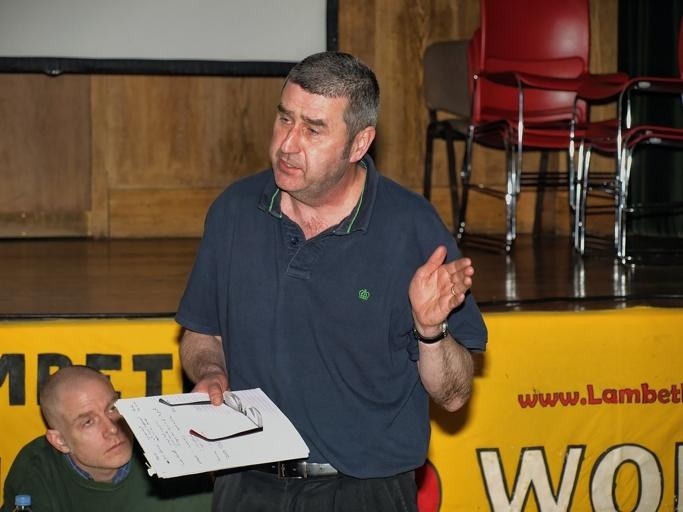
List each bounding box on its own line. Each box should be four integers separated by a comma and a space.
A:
159, 390, 263, 442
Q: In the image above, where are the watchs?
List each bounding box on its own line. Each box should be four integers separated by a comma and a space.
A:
410, 320, 451, 343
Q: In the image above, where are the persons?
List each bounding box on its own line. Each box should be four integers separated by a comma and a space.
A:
0, 365, 214, 512
174, 51, 488, 512
